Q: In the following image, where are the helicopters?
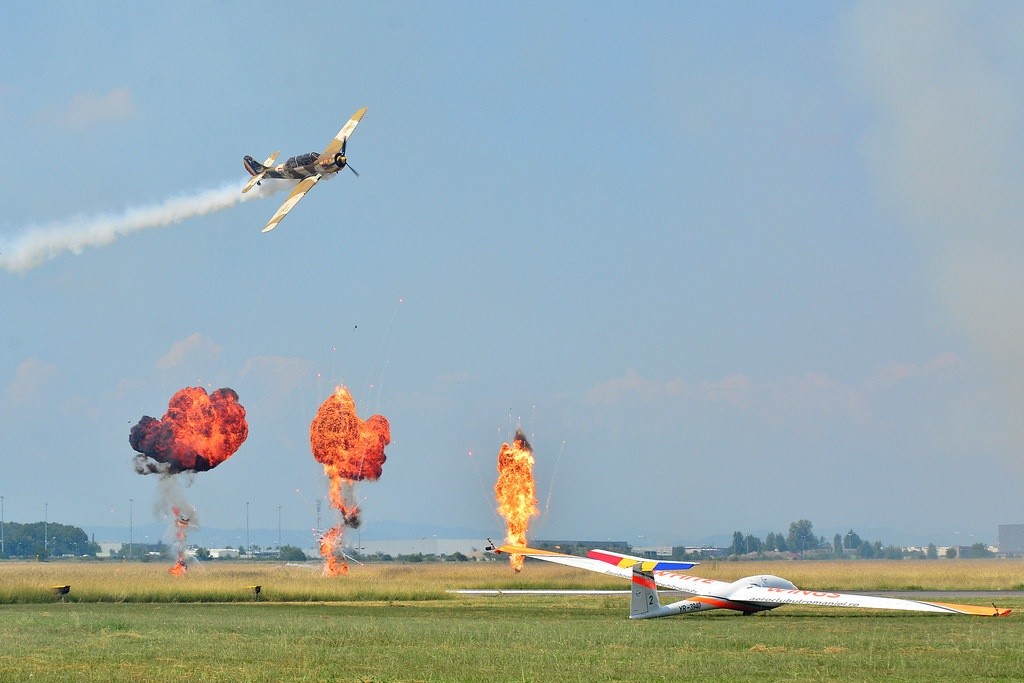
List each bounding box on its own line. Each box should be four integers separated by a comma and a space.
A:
240, 106, 371, 234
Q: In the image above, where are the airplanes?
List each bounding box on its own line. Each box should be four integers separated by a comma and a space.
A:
484, 535, 1023, 620
